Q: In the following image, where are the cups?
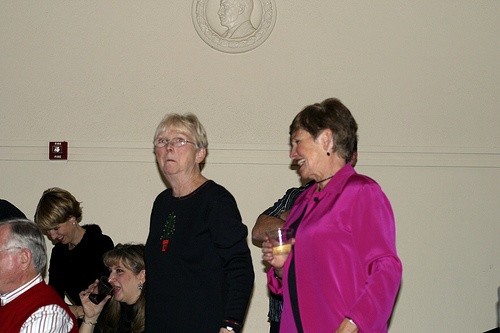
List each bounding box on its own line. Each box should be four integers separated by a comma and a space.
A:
265, 228, 294, 258
88, 276, 119, 306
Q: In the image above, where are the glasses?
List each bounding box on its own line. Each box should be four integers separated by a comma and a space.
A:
153, 137, 198, 148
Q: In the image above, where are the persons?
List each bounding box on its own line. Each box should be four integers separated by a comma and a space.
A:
0, 199, 79, 333
252, 98, 403, 333
145, 111, 255, 333
34, 187, 115, 328
78, 243, 145, 333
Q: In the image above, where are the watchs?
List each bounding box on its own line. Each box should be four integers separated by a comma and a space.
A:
221, 325, 236, 333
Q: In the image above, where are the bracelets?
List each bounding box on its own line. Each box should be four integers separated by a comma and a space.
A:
83, 319, 97, 325
345, 316, 359, 331
